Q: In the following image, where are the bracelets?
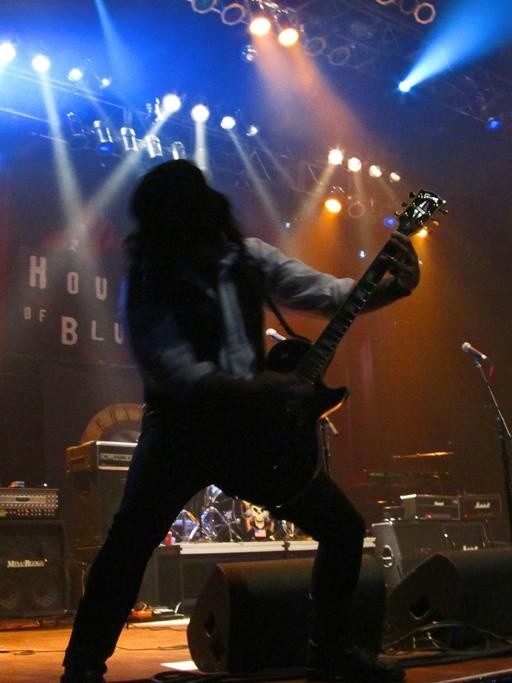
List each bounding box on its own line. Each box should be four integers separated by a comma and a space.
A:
381, 276, 411, 299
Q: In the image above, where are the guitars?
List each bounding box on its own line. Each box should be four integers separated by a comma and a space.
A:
211, 188, 451, 508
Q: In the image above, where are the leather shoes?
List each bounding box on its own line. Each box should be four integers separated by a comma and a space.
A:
303, 631, 406, 681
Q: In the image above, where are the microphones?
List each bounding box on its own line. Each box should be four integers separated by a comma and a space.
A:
462, 341, 487, 363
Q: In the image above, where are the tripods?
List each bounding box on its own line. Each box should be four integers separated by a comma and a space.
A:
188, 490, 241, 542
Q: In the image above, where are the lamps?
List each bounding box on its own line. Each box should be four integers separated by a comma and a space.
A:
62, 111, 188, 164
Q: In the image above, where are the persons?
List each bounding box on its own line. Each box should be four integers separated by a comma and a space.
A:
58, 156, 420, 683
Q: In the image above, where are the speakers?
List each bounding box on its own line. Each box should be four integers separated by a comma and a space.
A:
371, 520, 511, 591
0, 518, 71, 617
61, 473, 159, 609
187, 552, 386, 673
384, 545, 512, 648
